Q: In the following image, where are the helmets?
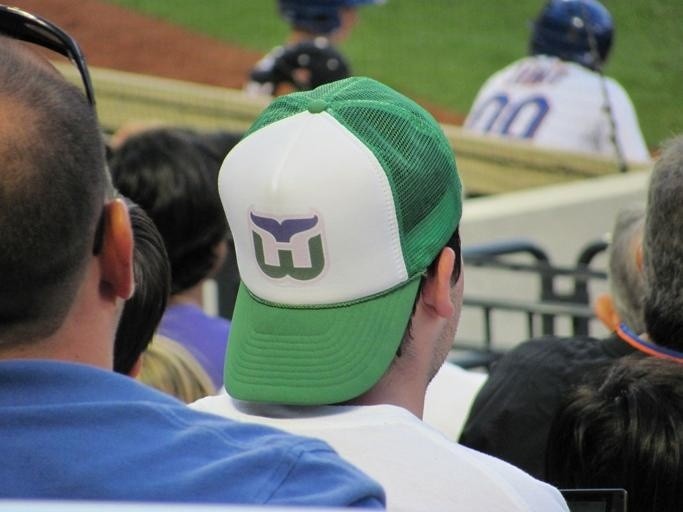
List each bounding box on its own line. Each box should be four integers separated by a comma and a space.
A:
277, 1, 383, 32
249, 39, 351, 91
529, 1, 614, 71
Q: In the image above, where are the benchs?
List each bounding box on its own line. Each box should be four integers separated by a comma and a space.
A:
50, 62, 651, 197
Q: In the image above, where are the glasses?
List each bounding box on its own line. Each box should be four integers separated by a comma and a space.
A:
0, 3, 106, 257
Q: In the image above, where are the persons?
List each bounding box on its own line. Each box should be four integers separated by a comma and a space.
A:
459, 2, 651, 169
465, 129, 682, 511
179, 70, 576, 511
595, 206, 651, 334
108, 120, 233, 392
0, 30, 390, 511
131, 335, 215, 403
247, 44, 351, 99
274, 3, 379, 49
108, 183, 177, 381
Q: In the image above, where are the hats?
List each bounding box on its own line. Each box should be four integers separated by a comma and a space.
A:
216, 76, 464, 407
